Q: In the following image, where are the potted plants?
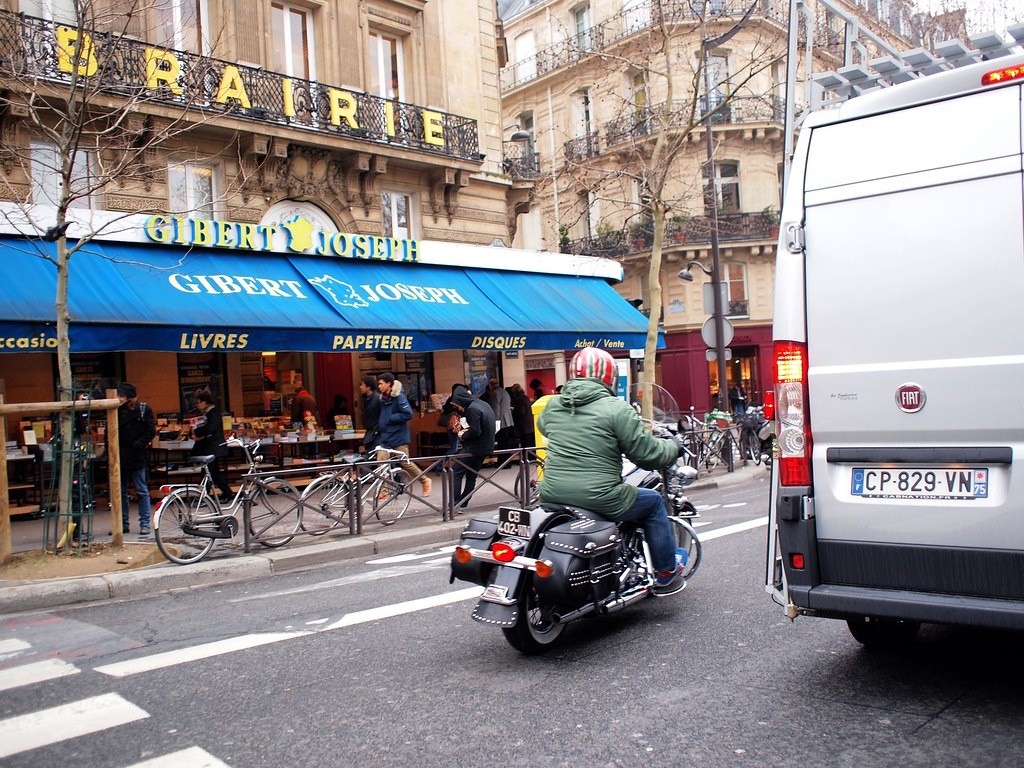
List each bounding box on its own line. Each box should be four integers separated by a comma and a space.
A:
673, 217, 686, 246
763, 204, 780, 238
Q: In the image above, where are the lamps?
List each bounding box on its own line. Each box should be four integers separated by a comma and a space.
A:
503, 123, 529, 143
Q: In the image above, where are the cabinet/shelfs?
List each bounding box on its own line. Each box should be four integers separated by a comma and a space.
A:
6, 454, 44, 515
144, 432, 373, 499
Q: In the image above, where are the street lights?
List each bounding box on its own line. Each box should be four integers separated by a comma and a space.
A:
677, 260, 734, 464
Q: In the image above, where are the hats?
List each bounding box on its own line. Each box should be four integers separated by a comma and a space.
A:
118, 382, 136, 397
71, 382, 83, 389
737, 382, 742, 387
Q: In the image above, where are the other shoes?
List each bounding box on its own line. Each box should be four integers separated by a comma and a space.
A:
447, 506, 465, 514
73, 532, 94, 542
220, 493, 235, 504
422, 479, 432, 497
395, 481, 406, 493
194, 500, 208, 507
365, 491, 388, 502
433, 466, 440, 476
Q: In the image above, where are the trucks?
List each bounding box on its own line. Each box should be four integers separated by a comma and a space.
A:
764, 21, 1024, 650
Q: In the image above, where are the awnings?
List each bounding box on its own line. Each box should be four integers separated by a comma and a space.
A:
0, 234, 668, 352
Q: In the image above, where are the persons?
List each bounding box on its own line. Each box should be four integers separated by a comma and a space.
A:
104, 383, 156, 535
326, 394, 350, 451
479, 378, 563, 468
51, 382, 95, 542
291, 385, 317, 458
354, 377, 405, 499
536, 346, 688, 587
366, 373, 432, 502
432, 384, 473, 476
448, 386, 496, 509
189, 392, 235, 508
728, 383, 747, 423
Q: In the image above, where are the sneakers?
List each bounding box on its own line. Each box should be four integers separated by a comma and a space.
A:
655, 547, 688, 587
109, 527, 130, 535
140, 524, 150, 534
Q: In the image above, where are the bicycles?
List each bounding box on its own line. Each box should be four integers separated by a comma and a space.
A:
647, 404, 764, 473
297, 445, 414, 536
152, 438, 303, 565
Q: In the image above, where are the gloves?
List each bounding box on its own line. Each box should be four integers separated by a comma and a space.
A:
132, 441, 143, 449
667, 434, 685, 451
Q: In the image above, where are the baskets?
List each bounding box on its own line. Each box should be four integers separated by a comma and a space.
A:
740, 413, 758, 428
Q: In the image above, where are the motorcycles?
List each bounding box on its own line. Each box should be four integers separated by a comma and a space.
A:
449, 415, 702, 654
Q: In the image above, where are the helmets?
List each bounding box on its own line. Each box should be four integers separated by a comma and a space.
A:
569, 346, 614, 386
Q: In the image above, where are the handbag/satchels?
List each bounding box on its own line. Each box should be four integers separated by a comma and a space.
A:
73, 440, 93, 465
446, 413, 461, 433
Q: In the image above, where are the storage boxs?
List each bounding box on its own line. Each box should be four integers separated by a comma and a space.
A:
450, 513, 500, 587
533, 518, 622, 605
